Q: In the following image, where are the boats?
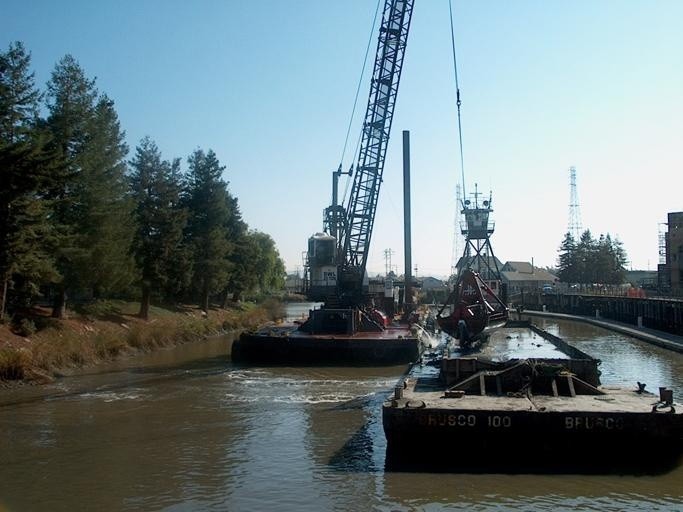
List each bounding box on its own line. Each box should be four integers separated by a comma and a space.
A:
382, 318, 683, 452
230, 293, 429, 364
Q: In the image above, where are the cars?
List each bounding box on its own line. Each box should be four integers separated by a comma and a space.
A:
542, 284, 552, 291
